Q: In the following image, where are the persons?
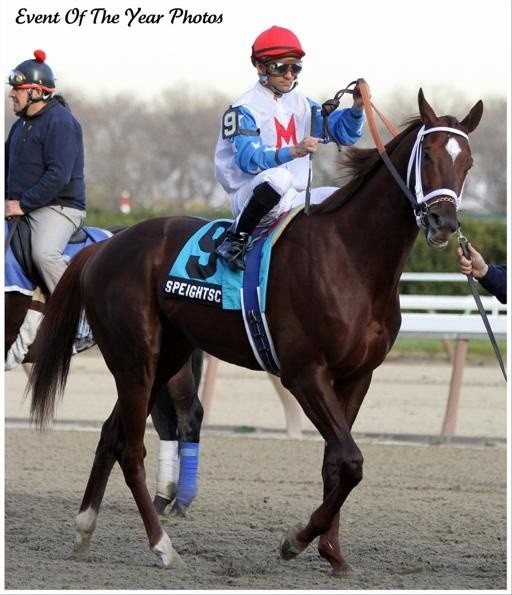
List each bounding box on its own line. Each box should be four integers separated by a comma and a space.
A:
452, 243, 506, 307
5, 49, 95, 353
214, 24, 371, 272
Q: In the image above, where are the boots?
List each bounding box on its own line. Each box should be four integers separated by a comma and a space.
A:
215, 231, 248, 270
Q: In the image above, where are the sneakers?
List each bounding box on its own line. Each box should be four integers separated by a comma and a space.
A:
74, 336, 94, 351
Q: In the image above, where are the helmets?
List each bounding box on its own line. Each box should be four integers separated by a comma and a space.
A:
252, 26, 305, 60
10, 51, 55, 91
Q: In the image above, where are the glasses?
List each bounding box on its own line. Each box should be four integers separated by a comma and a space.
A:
9, 70, 41, 85
254, 57, 302, 76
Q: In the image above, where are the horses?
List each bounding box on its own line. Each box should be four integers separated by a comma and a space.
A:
5, 221, 206, 524
23, 85, 484, 578
